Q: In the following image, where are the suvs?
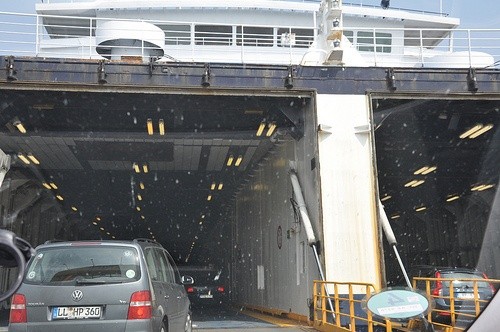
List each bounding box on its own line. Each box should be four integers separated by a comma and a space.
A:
7, 238, 193, 332
403, 264, 495, 322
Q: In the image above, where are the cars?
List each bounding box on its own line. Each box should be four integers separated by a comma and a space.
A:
181, 265, 231, 310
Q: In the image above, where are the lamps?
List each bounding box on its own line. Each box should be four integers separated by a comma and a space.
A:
12, 119, 279, 201
381, 123, 497, 219
